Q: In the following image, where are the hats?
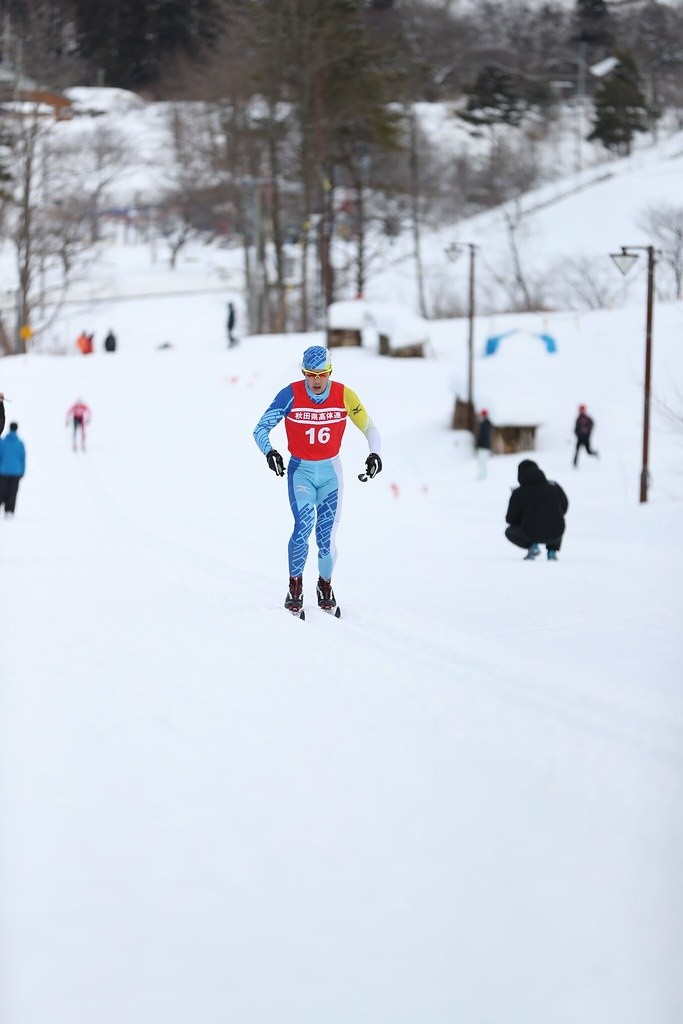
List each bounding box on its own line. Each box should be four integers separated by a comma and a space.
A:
303, 346, 331, 372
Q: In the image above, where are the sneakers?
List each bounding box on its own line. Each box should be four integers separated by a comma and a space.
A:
316, 575, 336, 608
284, 576, 303, 612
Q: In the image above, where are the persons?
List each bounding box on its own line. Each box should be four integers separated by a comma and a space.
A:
476, 411, 492, 449
253, 346, 383, 610
78, 332, 93, 354
20, 322, 32, 352
228, 304, 234, 342
574, 406, 599, 465
0, 393, 25, 519
67, 399, 91, 453
105, 334, 115, 351
505, 460, 568, 560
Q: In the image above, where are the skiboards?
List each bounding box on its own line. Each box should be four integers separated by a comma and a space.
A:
284, 598, 341, 621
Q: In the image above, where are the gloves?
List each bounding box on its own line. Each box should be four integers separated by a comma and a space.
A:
365, 453, 383, 479
266, 450, 286, 476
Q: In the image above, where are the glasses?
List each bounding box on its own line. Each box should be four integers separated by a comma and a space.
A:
303, 368, 331, 378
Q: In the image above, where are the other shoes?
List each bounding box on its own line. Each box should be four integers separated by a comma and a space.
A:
547, 550, 557, 560
524, 544, 540, 560
